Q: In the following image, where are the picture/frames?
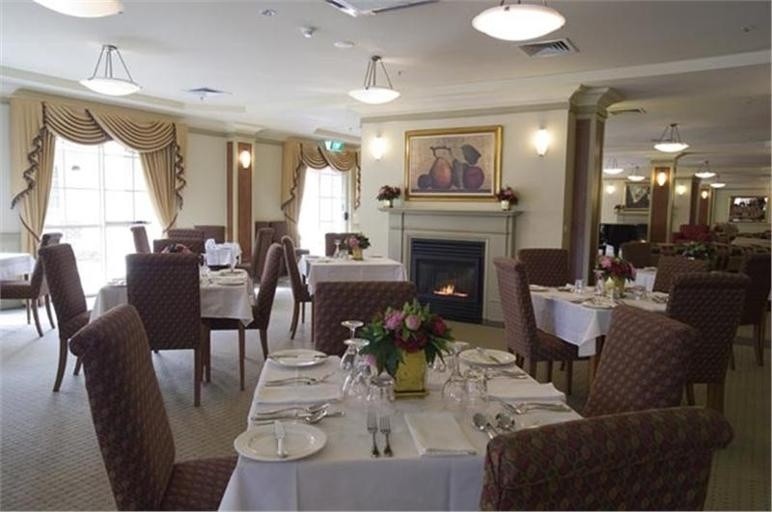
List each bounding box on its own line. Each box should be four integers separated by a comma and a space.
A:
402, 124, 503, 202
728, 195, 769, 225
624, 182, 651, 211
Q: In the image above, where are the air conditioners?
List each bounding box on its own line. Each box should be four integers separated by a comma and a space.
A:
514, 37, 579, 60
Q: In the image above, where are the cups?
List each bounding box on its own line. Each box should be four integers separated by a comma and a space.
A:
593, 269, 605, 296
372, 377, 395, 417
342, 338, 371, 409
441, 343, 469, 412
340, 322, 361, 372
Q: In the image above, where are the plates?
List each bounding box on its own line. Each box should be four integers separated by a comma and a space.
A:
270, 349, 327, 366
460, 349, 516, 364
582, 300, 624, 308
234, 423, 327, 461
522, 411, 579, 426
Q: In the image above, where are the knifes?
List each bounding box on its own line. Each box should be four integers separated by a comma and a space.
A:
272, 419, 288, 457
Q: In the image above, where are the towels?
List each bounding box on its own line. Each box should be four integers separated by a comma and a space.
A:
256, 383, 337, 404
403, 411, 477, 456
487, 381, 565, 401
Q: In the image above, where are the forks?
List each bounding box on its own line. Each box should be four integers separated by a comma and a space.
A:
500, 399, 559, 410
506, 405, 571, 415
264, 371, 336, 388
366, 412, 381, 458
379, 416, 393, 457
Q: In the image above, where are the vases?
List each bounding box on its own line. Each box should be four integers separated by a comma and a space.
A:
594, 272, 608, 297
393, 351, 428, 399
389, 200, 393, 208
353, 249, 363, 261
613, 280, 624, 298
501, 200, 510, 210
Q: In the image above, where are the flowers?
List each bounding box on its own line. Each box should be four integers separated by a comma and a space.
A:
346, 234, 371, 251
495, 187, 518, 205
375, 185, 400, 200
591, 256, 613, 271
602, 257, 637, 284
353, 297, 454, 379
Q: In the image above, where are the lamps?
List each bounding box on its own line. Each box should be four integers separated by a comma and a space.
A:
710, 174, 726, 189
602, 155, 624, 176
532, 127, 552, 158
470, 0, 566, 42
348, 56, 400, 106
653, 123, 690, 153
656, 168, 667, 187
239, 147, 252, 169
695, 160, 717, 178
371, 135, 387, 161
626, 166, 645, 183
79, 44, 143, 98
33, 1, 124, 19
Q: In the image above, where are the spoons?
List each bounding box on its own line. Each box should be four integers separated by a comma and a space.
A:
495, 412, 517, 434
472, 413, 493, 440
251, 409, 328, 423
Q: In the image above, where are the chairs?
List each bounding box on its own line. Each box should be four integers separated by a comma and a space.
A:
517, 247, 570, 290
125, 252, 211, 408
37, 243, 93, 392
215, 243, 283, 361
706, 242, 729, 270
667, 272, 750, 416
581, 303, 698, 416
492, 254, 591, 405
281, 235, 315, 344
730, 253, 771, 372
313, 280, 417, 354
240, 227, 275, 285
168, 230, 204, 254
620, 242, 650, 270
255, 221, 269, 238
194, 225, 224, 244
325, 233, 358, 256
0, 233, 63, 338
270, 221, 309, 256
131, 226, 150, 253
68, 303, 239, 512
479, 404, 735, 511
153, 238, 171, 254
651, 256, 705, 296
